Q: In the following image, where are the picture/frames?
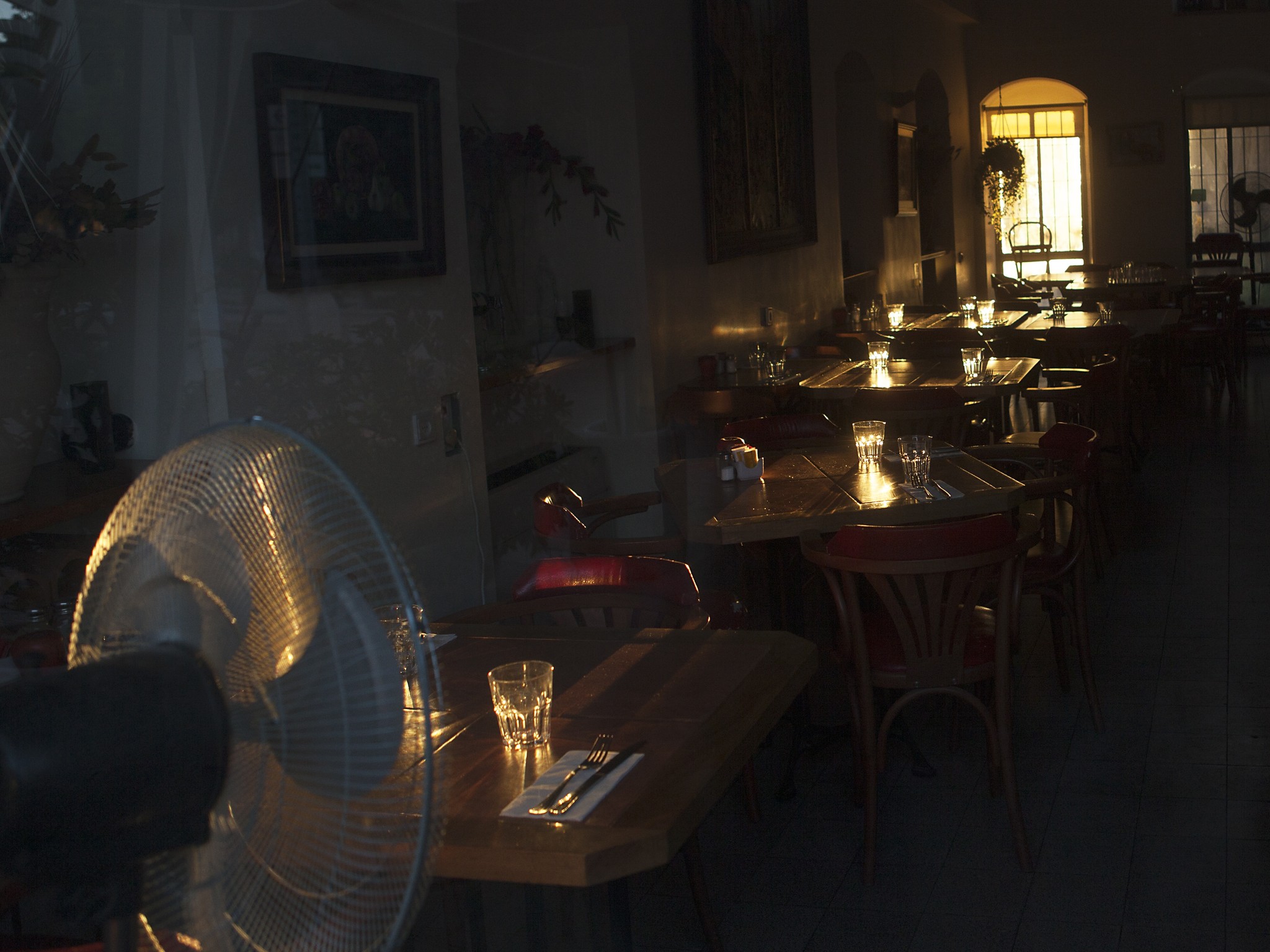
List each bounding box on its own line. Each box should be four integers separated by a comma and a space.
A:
254, 52, 446, 289
894, 119, 919, 218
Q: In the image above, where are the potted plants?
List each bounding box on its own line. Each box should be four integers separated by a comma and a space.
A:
1, 0, 164, 504
975, 135, 1025, 243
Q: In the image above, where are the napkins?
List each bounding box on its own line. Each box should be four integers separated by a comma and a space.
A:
500, 749, 645, 822
898, 480, 965, 502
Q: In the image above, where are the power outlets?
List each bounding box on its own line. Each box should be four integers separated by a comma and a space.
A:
413, 413, 435, 444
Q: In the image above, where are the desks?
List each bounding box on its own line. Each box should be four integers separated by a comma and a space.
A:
682, 357, 1040, 396
652, 435, 1025, 546
1013, 310, 1123, 332
834, 313, 928, 338
1066, 271, 1112, 296
1023, 272, 1083, 286
234, 619, 817, 889
913, 310, 1028, 332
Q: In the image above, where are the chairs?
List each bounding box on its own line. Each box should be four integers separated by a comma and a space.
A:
1009, 222, 1052, 278
504, 266, 1254, 951
1196, 233, 1243, 260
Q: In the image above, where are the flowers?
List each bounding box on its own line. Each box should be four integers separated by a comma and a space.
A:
460, 123, 624, 332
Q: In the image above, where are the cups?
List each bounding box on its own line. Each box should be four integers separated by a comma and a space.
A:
486, 660, 555, 750
1107, 260, 1163, 283
370, 603, 424, 678
852, 420, 886, 463
865, 299, 882, 320
976, 300, 994, 319
958, 297, 976, 317
1048, 297, 1067, 320
749, 353, 764, 370
898, 435, 933, 480
765, 360, 785, 378
961, 348, 985, 376
886, 303, 905, 325
1096, 301, 1114, 324
867, 342, 890, 368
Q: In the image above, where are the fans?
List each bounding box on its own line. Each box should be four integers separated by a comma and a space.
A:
0, 416, 448, 952
1220, 171, 1270, 306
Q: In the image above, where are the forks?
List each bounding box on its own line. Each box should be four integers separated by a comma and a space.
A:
909, 473, 937, 500
979, 369, 994, 383
992, 314, 1000, 325
770, 369, 791, 383
527, 734, 614, 815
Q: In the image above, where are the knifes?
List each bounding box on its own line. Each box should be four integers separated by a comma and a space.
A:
988, 374, 999, 383
548, 738, 650, 815
926, 479, 951, 498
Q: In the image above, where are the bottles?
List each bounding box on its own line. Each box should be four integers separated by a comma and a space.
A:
712, 451, 734, 482
725, 354, 737, 374
851, 304, 861, 324
